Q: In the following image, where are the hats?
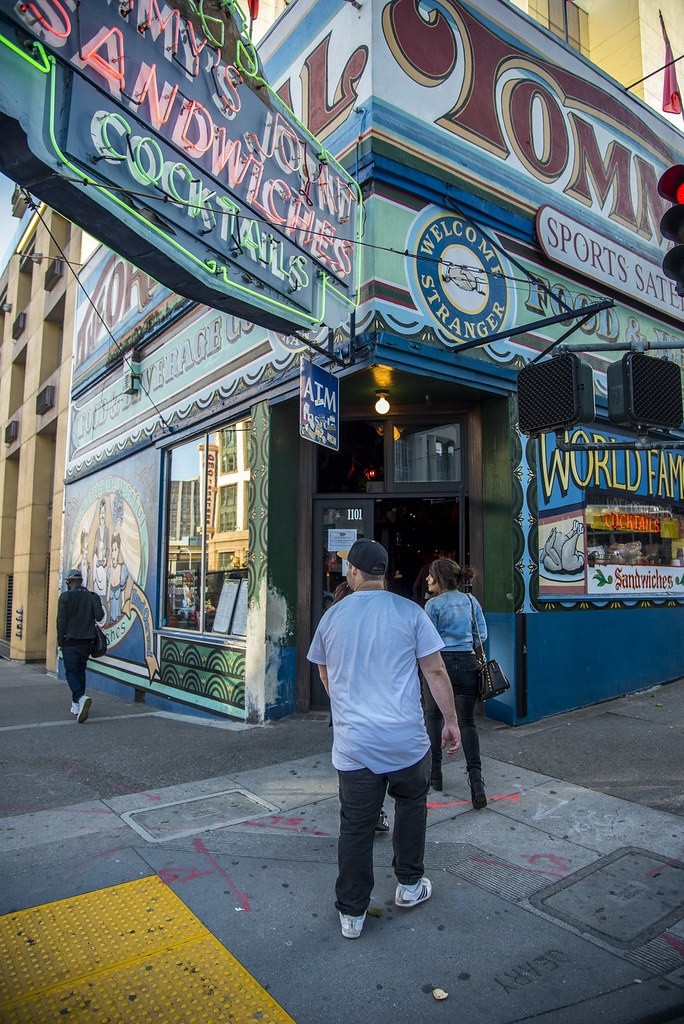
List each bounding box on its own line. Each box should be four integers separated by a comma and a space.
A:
336, 538, 388, 576
63, 569, 83, 580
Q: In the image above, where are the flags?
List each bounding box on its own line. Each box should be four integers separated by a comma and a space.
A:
663, 26, 682, 114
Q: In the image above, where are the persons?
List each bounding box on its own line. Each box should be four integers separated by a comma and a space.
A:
421, 557, 488, 809
56, 569, 105, 722
306, 540, 461, 938
327, 581, 390, 831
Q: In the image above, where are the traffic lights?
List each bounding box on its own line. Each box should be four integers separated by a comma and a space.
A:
658, 164, 684, 298
607, 353, 682, 427
516, 353, 596, 435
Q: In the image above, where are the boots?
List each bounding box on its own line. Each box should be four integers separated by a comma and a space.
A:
466, 768, 487, 811
429, 778, 442, 791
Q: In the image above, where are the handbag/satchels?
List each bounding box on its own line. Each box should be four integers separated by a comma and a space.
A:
479, 659, 510, 703
91, 625, 108, 658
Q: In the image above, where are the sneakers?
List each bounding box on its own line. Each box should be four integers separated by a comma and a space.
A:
77, 695, 92, 723
394, 878, 433, 907
373, 811, 390, 830
70, 702, 80, 714
338, 908, 366, 938
